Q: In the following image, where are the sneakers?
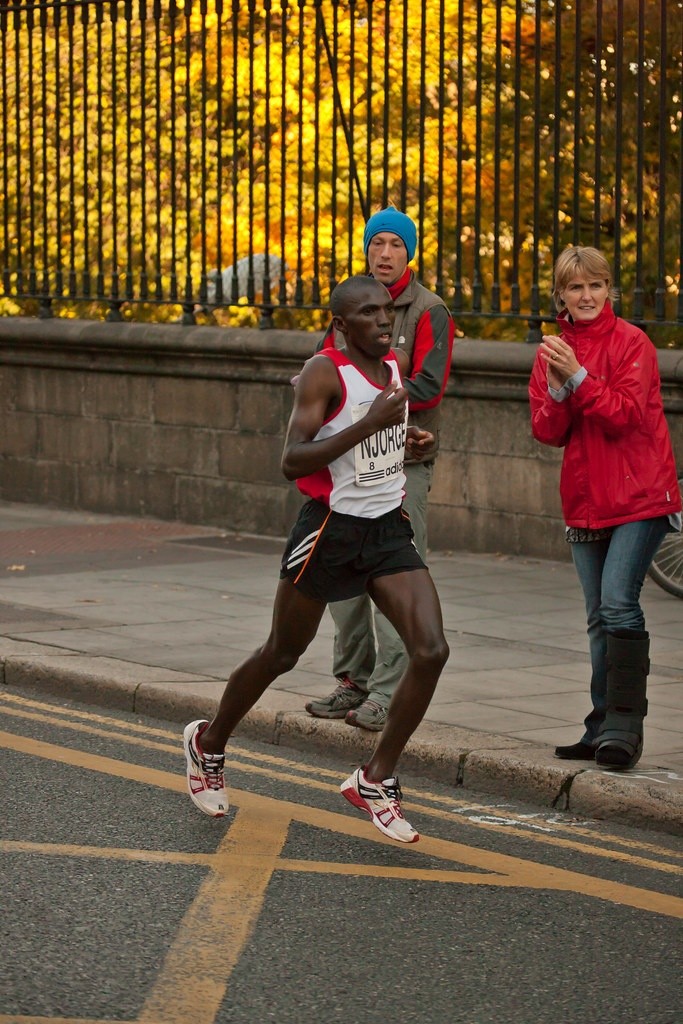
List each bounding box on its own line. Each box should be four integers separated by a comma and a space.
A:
305, 677, 368, 719
340, 764, 420, 843
183, 719, 229, 818
345, 699, 390, 731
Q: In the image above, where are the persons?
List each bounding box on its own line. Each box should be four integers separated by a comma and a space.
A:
528, 245, 683, 771
183, 276, 453, 843
289, 206, 457, 732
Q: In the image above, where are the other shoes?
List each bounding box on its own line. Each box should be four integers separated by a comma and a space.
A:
597, 746, 631, 770
554, 741, 594, 759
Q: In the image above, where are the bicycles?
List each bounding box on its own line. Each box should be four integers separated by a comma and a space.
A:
646, 470, 683, 600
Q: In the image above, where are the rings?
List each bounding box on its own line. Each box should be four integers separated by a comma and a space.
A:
552, 354, 559, 359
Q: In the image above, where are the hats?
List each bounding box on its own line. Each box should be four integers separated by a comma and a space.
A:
363, 206, 417, 264
